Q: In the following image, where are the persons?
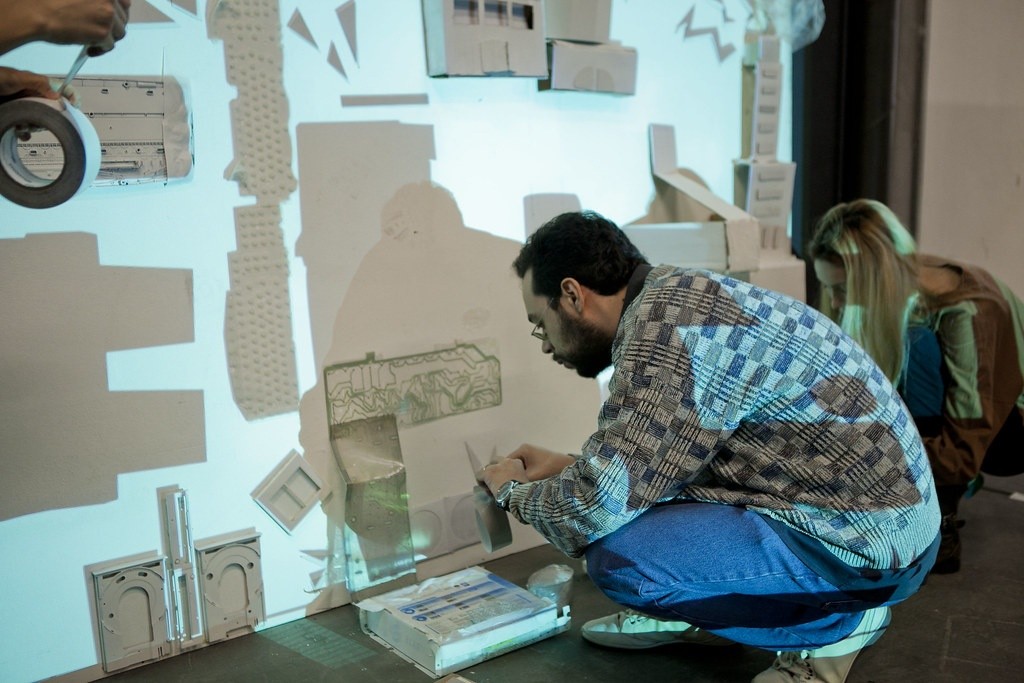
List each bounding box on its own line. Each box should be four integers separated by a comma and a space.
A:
474, 211, 941, 683
0, 0, 130, 141
809, 199, 1024, 576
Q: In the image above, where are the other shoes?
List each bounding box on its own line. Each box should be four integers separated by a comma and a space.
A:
751, 606, 892, 682
581, 607, 740, 648
936, 512, 964, 574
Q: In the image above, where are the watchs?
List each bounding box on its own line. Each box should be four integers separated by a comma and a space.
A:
495, 479, 526, 512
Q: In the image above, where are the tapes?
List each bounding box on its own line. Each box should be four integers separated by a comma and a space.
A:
473, 486, 514, 554
0, 45, 102, 211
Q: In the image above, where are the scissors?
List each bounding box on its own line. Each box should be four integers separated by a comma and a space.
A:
464, 441, 497, 497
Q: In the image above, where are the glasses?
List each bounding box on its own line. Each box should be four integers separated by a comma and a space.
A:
529, 296, 553, 341
